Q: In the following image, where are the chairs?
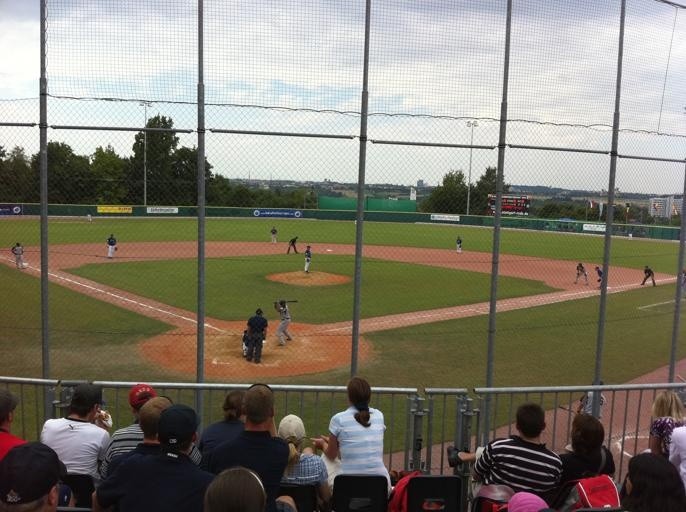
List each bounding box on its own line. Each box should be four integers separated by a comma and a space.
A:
54, 470, 96, 511
269, 480, 318, 512
405, 475, 464, 512
331, 473, 389, 512
469, 479, 519, 511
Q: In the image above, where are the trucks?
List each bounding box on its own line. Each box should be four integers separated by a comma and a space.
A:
553, 191, 571, 201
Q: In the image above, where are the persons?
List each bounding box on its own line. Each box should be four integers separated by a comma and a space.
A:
241, 301, 292, 363
455, 236, 462, 254
105, 234, 117, 259
595, 266, 603, 289
11, 242, 27, 269
641, 265, 658, 286
287, 236, 299, 254
1, 379, 393, 511
304, 246, 311, 272
575, 263, 589, 285
87, 213, 92, 223
269, 226, 278, 243
448, 386, 686, 512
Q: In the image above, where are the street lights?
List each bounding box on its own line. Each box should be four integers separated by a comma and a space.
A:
466, 120, 479, 215
139, 99, 152, 206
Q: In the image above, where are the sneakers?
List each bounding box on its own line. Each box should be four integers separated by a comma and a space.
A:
447, 446, 463, 467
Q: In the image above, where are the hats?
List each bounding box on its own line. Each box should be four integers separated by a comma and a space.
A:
278, 414, 305, 443
129, 384, 157, 407
71, 383, 105, 405
158, 404, 201, 446
0, 388, 18, 414
0, 441, 67, 504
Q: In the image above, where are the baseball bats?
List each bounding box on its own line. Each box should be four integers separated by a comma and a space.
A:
558, 404, 577, 414
274, 300, 297, 303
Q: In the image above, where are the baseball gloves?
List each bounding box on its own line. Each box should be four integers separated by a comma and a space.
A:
597, 278, 601, 282
114, 246, 118, 251
